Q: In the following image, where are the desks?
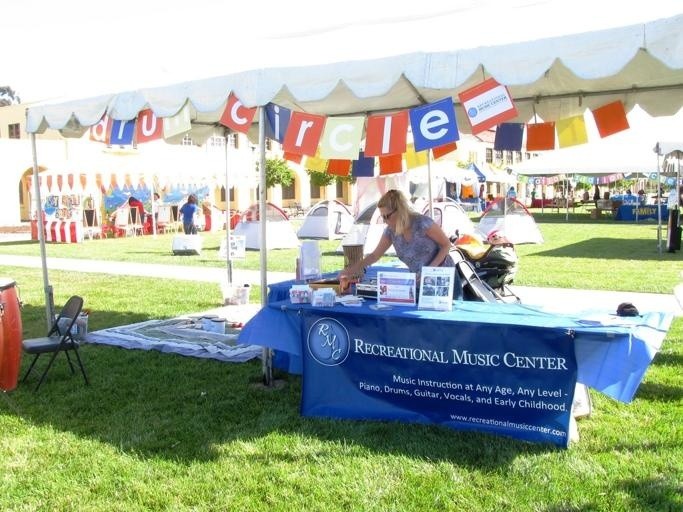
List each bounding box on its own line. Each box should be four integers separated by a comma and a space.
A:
614, 204, 668, 223
266, 265, 417, 375
236, 297, 653, 449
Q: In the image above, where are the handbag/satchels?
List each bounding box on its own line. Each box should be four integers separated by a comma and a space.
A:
616, 303, 642, 318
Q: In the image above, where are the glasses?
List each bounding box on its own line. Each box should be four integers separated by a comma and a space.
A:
383, 209, 397, 219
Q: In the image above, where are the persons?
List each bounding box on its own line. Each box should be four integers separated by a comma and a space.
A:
593, 182, 646, 208
347, 189, 518, 303
507, 187, 516, 198
531, 188, 537, 200
129, 192, 198, 235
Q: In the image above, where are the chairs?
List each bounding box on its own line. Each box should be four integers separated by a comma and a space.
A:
21, 294, 88, 390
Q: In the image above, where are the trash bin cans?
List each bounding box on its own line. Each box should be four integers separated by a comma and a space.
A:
220, 283, 251, 306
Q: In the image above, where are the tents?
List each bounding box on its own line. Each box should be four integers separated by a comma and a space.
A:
297, 198, 356, 241
233, 200, 301, 250
478, 196, 542, 244
335, 193, 485, 256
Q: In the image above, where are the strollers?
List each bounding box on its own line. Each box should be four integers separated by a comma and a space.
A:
447, 228, 522, 304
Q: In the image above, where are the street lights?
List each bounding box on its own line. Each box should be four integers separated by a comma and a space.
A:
652, 142, 663, 256
